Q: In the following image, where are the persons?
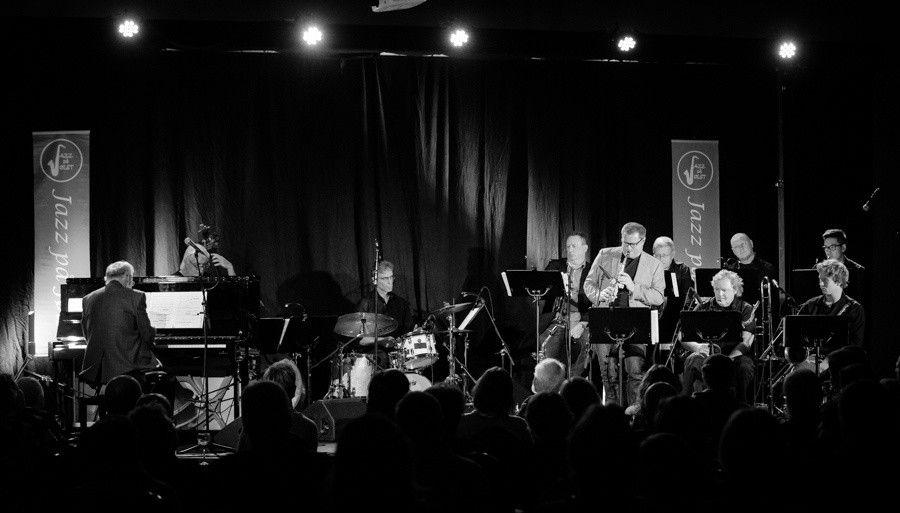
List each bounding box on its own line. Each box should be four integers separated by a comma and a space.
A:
79, 260, 177, 426
336, 342, 900, 513
352, 261, 410, 370
539, 222, 870, 414
171, 243, 237, 276
0, 359, 319, 513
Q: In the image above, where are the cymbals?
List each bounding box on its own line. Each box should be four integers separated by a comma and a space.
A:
333, 312, 398, 338
422, 301, 482, 321
432, 326, 472, 333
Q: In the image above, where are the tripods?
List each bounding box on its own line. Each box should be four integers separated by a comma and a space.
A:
178, 250, 238, 454
309, 321, 366, 400
441, 313, 473, 403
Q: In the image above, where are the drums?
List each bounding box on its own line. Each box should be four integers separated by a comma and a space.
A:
329, 349, 376, 403
395, 324, 439, 370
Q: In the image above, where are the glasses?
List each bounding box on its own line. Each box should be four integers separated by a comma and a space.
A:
656, 254, 671, 259
381, 276, 395, 280
131, 282, 134, 284
822, 244, 841, 251
623, 239, 642, 249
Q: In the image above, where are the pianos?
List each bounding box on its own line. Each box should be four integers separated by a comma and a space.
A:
47, 273, 262, 434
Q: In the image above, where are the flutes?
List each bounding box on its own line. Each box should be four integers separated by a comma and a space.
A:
608, 255, 628, 302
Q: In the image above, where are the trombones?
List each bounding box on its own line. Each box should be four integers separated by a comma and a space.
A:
760, 274, 780, 418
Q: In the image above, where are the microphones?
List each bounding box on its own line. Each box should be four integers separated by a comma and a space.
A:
599, 264, 616, 285
185, 238, 205, 256
422, 315, 432, 328
376, 242, 381, 259
778, 286, 796, 304
473, 288, 484, 308
14, 354, 33, 382
863, 188, 880, 211
690, 287, 703, 305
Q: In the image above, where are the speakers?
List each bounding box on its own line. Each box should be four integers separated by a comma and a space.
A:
303, 397, 367, 442
